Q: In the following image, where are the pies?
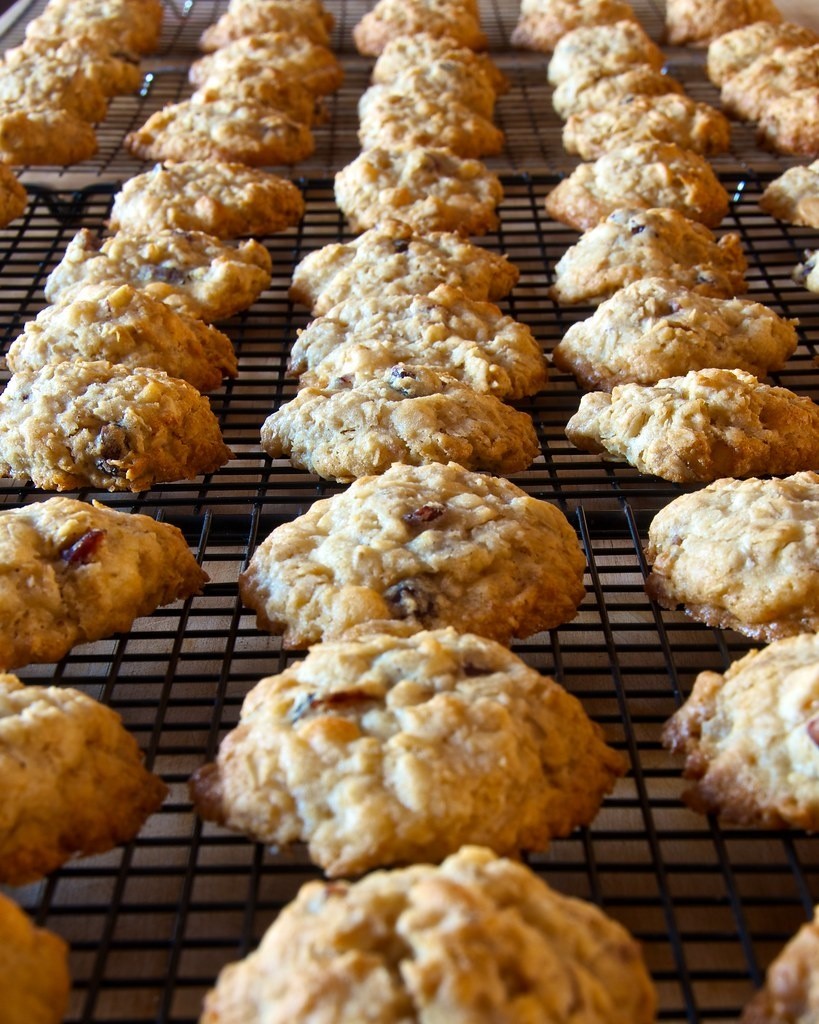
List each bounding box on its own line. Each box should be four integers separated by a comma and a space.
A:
0, 0, 819, 1024
